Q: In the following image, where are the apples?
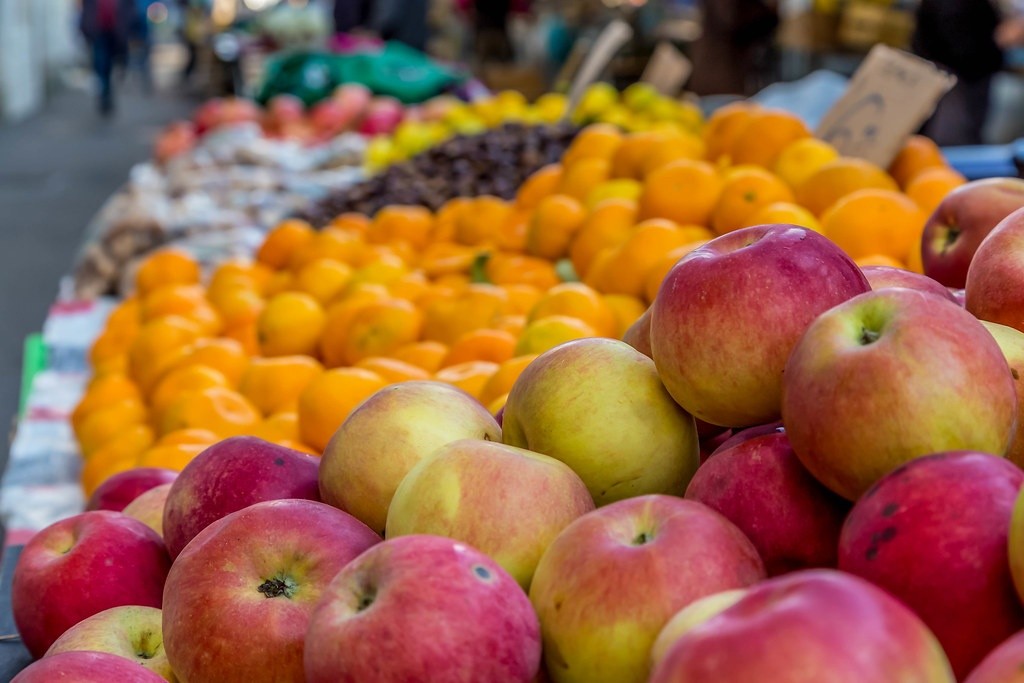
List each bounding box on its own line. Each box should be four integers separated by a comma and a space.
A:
0, 177, 1024, 683
144, 79, 455, 152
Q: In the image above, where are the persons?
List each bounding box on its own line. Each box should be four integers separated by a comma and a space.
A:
78, 0, 131, 119
913, 0, 1024, 147
331, 0, 519, 67
705, 1, 783, 91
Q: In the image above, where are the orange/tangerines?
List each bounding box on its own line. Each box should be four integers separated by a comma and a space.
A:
70, 97, 962, 497
